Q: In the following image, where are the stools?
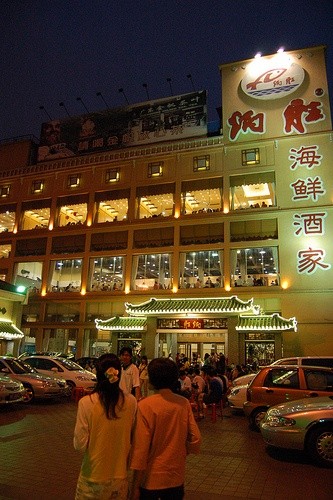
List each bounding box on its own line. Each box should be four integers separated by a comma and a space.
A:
70, 386, 86, 408
189, 392, 228, 423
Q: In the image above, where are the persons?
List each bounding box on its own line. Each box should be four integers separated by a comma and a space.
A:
66, 221, 87, 227
92, 282, 124, 291
51, 286, 61, 293
248, 201, 268, 209
248, 345, 254, 364
120, 347, 142, 403
191, 207, 221, 214
154, 281, 171, 290
138, 356, 149, 397
73, 353, 137, 500
183, 276, 221, 289
168, 351, 260, 419
113, 215, 118, 222
128, 358, 201, 500
32, 224, 48, 231
234, 274, 278, 287
23, 285, 39, 293
66, 283, 79, 293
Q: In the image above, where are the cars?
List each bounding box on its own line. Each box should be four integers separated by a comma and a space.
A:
0, 356, 68, 403
231, 356, 333, 386
0, 375, 27, 403
226, 384, 248, 409
243, 365, 333, 432
259, 396, 333, 461
20, 355, 95, 398
